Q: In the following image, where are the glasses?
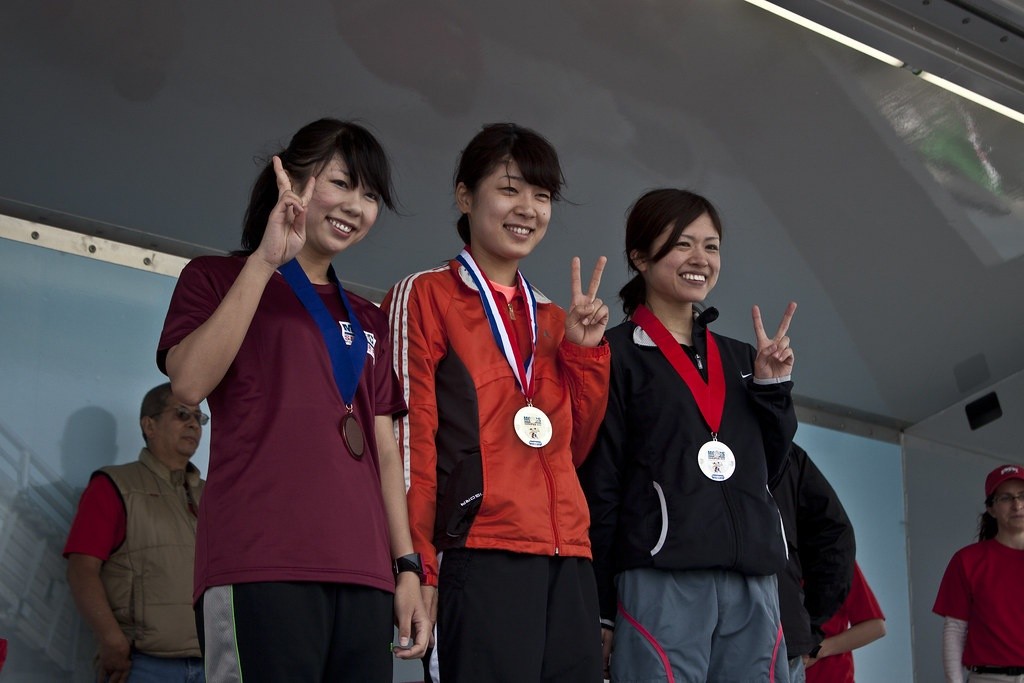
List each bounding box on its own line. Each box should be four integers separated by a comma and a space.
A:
993, 494, 1024, 504
151, 410, 209, 426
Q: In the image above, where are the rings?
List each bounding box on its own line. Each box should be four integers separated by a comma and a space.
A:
601, 643, 603, 647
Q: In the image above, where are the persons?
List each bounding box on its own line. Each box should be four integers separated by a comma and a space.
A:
379, 122, 611, 683
578, 189, 799, 683
62, 381, 205, 683
932, 464, 1024, 683
773, 440, 887, 683
156, 118, 431, 683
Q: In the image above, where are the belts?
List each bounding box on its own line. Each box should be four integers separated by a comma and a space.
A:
968, 666, 1024, 676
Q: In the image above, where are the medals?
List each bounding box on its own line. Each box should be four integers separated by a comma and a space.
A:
514, 404, 553, 448
342, 414, 366, 459
698, 438, 735, 481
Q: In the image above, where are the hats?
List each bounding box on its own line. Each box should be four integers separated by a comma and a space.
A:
985, 465, 1024, 496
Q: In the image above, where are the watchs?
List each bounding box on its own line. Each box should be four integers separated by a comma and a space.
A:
393, 552, 427, 586
